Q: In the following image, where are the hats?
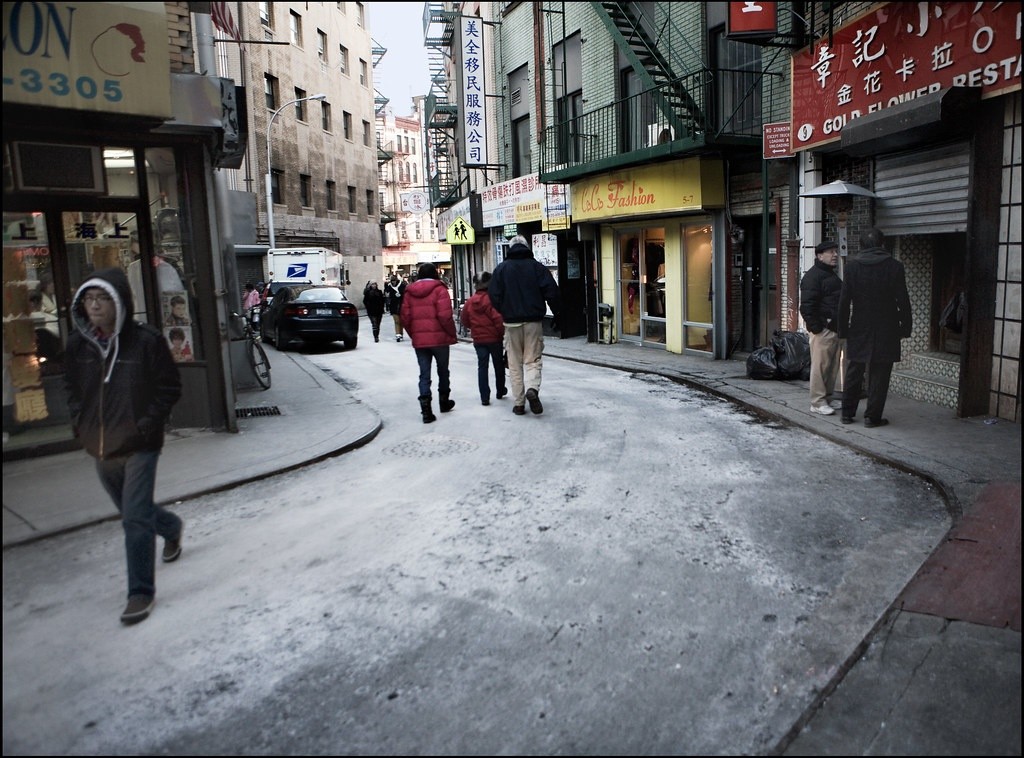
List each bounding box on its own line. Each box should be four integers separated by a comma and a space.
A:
474, 271, 491, 290
815, 242, 837, 255
508, 236, 528, 249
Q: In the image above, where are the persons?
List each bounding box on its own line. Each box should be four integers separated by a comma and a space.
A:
166, 296, 190, 326
839, 227, 913, 427
242, 281, 266, 340
17, 278, 77, 339
651, 250, 665, 343
168, 328, 194, 362
462, 271, 507, 406
488, 234, 565, 416
800, 241, 845, 416
384, 272, 419, 343
363, 280, 384, 342
65, 267, 183, 625
400, 264, 455, 423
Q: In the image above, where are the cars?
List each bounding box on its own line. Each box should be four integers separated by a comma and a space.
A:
259, 284, 358, 350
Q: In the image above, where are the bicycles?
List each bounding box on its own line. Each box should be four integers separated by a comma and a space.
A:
230, 299, 273, 388
452, 298, 471, 337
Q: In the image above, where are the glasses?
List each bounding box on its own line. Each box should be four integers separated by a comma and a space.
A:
80, 295, 110, 305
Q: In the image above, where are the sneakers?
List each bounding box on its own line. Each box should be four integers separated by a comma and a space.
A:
161, 520, 183, 562
120, 596, 156, 622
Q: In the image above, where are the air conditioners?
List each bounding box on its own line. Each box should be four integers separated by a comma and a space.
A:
647, 122, 676, 148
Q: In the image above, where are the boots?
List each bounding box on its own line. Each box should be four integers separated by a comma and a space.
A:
418, 395, 437, 424
440, 389, 456, 413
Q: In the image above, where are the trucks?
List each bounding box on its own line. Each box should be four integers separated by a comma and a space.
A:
267, 247, 349, 292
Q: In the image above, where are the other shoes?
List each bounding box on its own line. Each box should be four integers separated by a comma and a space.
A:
512, 406, 524, 415
840, 415, 852, 424
865, 417, 888, 428
810, 404, 834, 415
497, 387, 507, 399
526, 389, 543, 414
829, 400, 843, 409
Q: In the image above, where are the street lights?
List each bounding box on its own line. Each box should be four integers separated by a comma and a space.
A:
266, 94, 326, 249
